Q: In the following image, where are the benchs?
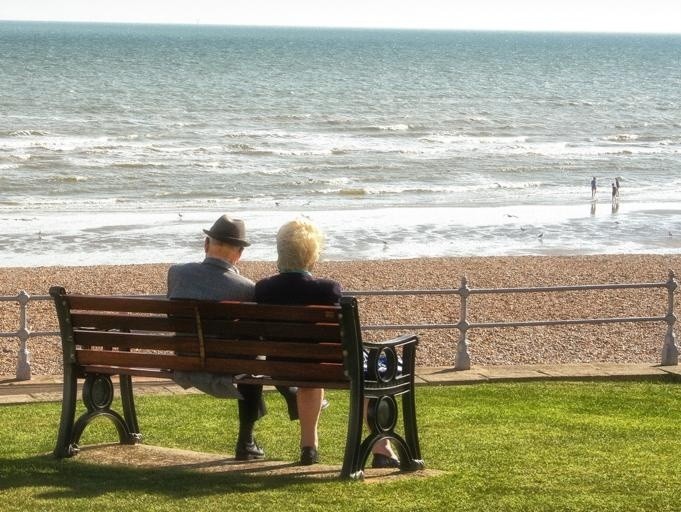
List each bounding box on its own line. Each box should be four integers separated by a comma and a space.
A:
48, 285, 424, 482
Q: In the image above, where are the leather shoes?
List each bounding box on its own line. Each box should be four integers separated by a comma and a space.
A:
288, 398, 329, 419
236, 439, 266, 460
300, 446, 318, 465
372, 453, 400, 468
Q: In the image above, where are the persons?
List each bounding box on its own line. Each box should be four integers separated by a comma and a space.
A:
254, 220, 401, 469
611, 182, 618, 200
615, 177, 621, 196
591, 177, 598, 199
163, 215, 333, 459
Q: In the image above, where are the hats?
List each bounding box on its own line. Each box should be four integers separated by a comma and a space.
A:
203, 214, 251, 247
